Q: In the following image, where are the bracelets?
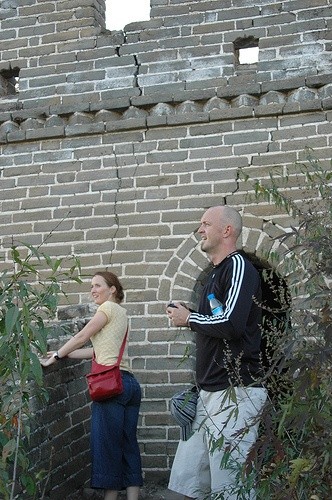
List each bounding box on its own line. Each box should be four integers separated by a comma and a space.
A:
185, 313, 192, 327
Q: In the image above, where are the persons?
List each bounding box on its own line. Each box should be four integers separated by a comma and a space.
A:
168, 206, 267, 500
39, 272, 143, 500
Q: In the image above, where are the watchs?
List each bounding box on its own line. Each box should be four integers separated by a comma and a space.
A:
53, 352, 61, 361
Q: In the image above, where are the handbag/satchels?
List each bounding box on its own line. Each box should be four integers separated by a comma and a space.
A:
86, 360, 123, 400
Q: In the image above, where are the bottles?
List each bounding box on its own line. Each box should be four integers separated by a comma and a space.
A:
208, 293, 225, 317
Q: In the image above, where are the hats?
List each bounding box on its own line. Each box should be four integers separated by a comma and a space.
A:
169, 389, 197, 441
